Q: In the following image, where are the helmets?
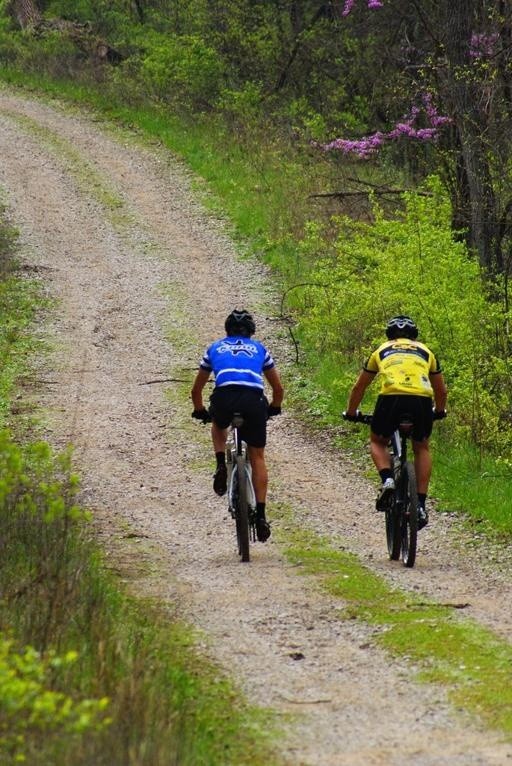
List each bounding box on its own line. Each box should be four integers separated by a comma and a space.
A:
386, 316, 418, 339
225, 309, 255, 333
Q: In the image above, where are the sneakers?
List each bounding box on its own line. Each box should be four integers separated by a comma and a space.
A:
376, 477, 396, 511
213, 464, 227, 496
256, 518, 270, 541
417, 506, 428, 531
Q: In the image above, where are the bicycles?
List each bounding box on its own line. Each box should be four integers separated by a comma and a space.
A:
336, 405, 451, 568
195, 400, 284, 566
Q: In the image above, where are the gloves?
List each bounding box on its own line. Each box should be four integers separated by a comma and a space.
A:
193, 408, 209, 424
268, 403, 281, 416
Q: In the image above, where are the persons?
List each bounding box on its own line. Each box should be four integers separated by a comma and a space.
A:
347, 317, 446, 531
192, 309, 284, 542
